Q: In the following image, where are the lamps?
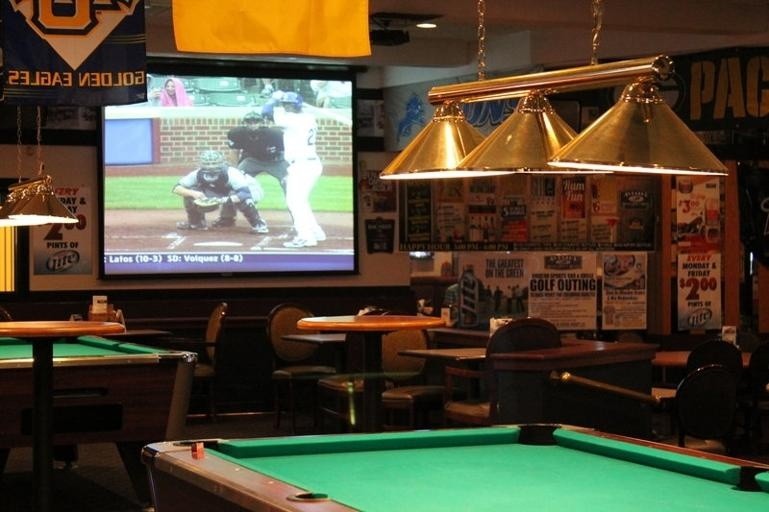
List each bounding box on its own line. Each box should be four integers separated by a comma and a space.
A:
377, 0, 728, 180
0, 101, 79, 227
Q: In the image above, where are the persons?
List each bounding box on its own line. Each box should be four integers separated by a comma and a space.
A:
148, 78, 186, 106
621, 253, 636, 272
311, 80, 352, 110
172, 150, 269, 234
484, 284, 525, 314
261, 90, 327, 248
212, 112, 293, 229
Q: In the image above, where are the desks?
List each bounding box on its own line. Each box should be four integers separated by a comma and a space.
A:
400, 344, 487, 404
0, 321, 124, 510
298, 315, 444, 417
2, 337, 199, 511
140, 421, 769, 512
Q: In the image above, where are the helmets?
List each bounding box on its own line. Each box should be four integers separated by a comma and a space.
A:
279, 91, 304, 110
240, 111, 266, 124
199, 149, 228, 176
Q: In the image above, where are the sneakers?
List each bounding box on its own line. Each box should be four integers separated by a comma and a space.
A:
174, 220, 209, 232
248, 223, 270, 233
211, 216, 236, 229
281, 234, 315, 249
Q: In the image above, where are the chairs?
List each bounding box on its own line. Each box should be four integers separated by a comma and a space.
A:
377, 310, 466, 430
263, 302, 335, 431
163, 301, 227, 423
555, 364, 735, 455
319, 305, 385, 434
748, 339, 768, 447
670, 339, 742, 374
452, 316, 559, 428
